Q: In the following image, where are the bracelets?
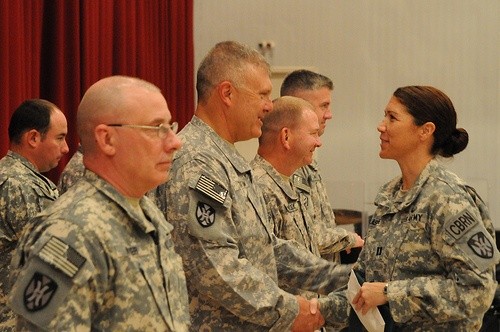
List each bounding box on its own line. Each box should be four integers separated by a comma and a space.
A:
382, 283, 389, 302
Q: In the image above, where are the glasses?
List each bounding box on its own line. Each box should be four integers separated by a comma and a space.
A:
107, 121, 178, 138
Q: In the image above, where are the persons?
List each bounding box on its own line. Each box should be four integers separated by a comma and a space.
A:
4, 75, 193, 332
144, 41, 326, 332
310, 84, 500, 332
249, 95, 322, 268
279, 69, 366, 263
2, 97, 73, 332
57, 141, 84, 197
